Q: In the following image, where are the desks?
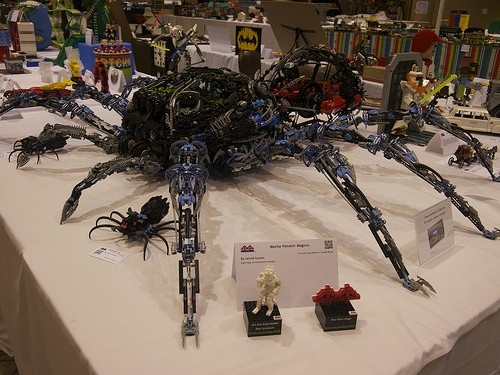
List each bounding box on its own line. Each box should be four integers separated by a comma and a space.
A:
160, 13, 283, 54
0, 51, 500, 375
178, 41, 383, 100
325, 29, 500, 85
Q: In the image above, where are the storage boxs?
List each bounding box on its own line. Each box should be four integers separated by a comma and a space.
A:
4, 56, 25, 74
8, 20, 38, 57
27, 61, 40, 67
0, 46, 10, 63
315, 301, 358, 333
243, 300, 282, 337
78, 42, 136, 76
362, 66, 387, 84
